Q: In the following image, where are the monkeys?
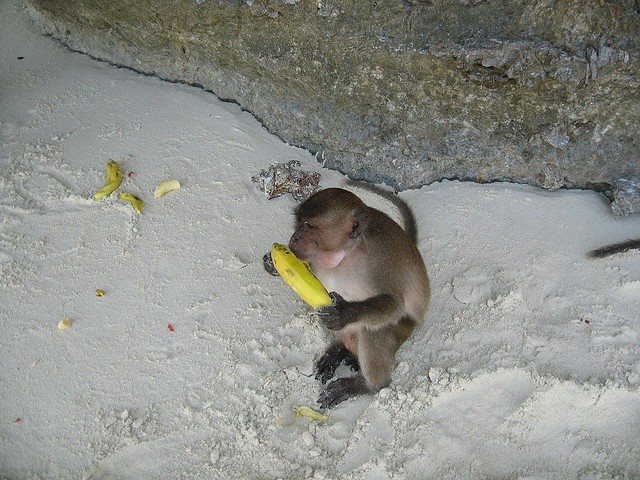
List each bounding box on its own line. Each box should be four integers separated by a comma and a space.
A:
258, 173, 433, 413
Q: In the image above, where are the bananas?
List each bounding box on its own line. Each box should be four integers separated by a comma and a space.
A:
270, 243, 331, 311
154, 179, 180, 199
